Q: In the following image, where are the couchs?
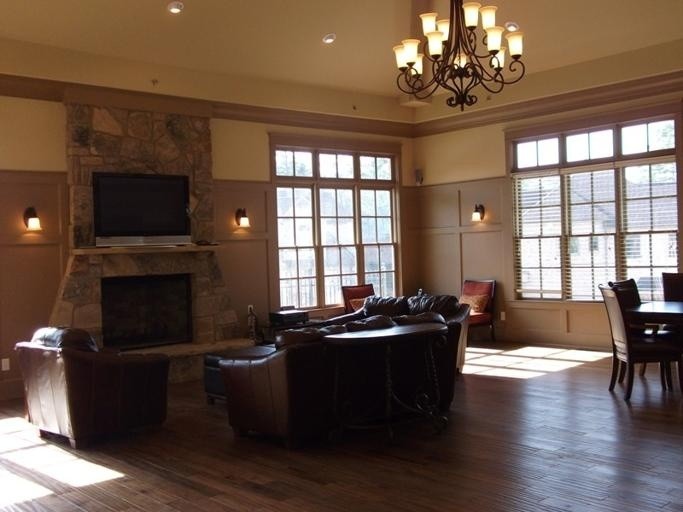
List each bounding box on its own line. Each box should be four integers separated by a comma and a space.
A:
12, 326, 170, 450
202, 294, 471, 452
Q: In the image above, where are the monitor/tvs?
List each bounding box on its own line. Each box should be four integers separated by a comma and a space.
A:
92, 172, 191, 248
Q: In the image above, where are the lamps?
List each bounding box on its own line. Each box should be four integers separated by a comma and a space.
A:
232, 207, 251, 228
23, 205, 42, 232
392, 0, 526, 112
471, 203, 485, 223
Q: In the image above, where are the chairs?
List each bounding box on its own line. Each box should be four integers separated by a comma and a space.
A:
596, 272, 682, 401
454, 279, 496, 346
12, 326, 170, 450
341, 283, 374, 313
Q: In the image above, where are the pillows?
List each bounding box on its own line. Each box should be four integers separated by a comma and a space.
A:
459, 294, 490, 313
